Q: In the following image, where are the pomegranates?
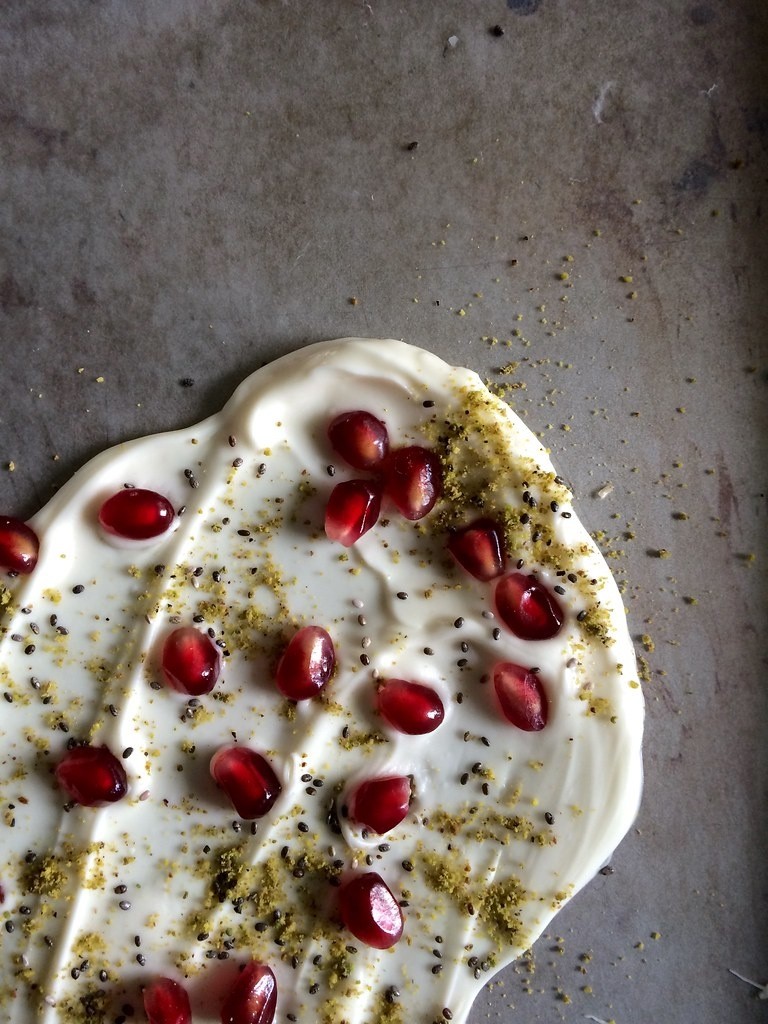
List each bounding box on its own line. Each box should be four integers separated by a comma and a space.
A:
0, 409, 564, 1024
